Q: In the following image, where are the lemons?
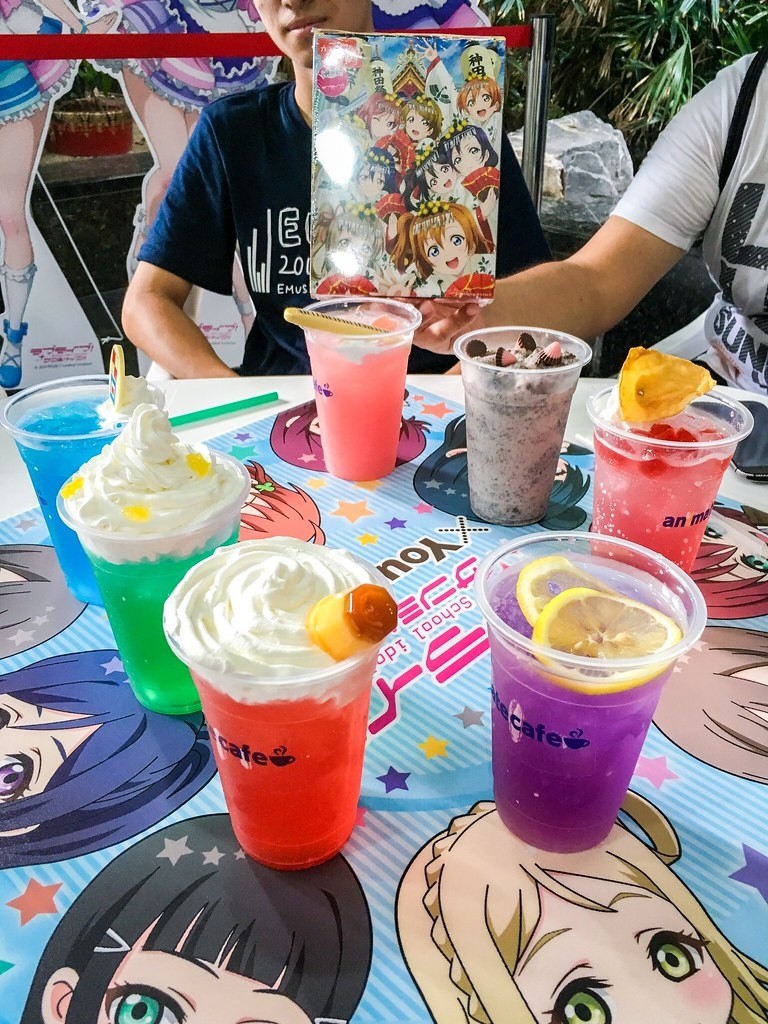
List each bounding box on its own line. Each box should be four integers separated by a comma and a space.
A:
531, 586, 684, 695
514, 553, 643, 648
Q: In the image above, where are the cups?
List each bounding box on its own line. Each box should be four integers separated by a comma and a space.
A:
161, 549, 386, 871
451, 325, 594, 527
585, 384, 755, 593
55, 447, 250, 716
473, 532, 708, 854
0, 374, 165, 605
298, 297, 423, 482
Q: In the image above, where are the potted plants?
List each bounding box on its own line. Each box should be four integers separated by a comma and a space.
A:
48, 62, 132, 159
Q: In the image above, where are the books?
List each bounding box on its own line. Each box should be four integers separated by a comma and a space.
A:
310, 29, 507, 301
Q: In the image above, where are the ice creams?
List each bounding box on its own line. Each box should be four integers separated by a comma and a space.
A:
98, 345, 163, 429
159, 533, 402, 706
57, 402, 249, 562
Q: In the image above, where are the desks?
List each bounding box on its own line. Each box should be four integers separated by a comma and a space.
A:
0, 373, 768, 1024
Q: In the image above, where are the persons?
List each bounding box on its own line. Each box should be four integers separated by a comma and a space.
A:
370, 49, 768, 396
119, 0, 553, 380
